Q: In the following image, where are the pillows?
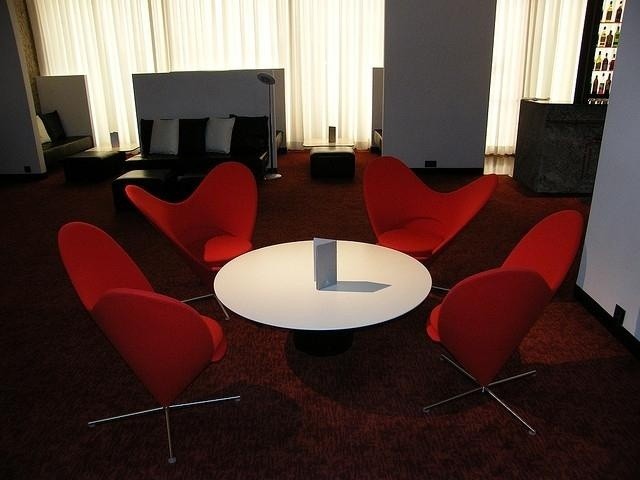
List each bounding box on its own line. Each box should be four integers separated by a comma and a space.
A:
40, 110, 64, 145
205, 116, 235, 154
149, 119, 179, 154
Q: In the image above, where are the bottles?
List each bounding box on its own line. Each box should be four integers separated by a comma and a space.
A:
590, 0, 624, 95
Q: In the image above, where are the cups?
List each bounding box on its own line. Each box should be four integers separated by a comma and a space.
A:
109, 132, 119, 148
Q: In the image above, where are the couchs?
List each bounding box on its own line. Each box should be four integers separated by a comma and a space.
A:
124, 114, 267, 169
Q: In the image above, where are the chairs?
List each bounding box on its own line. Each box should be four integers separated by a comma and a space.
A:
362, 157, 499, 292
57, 220, 242, 463
422, 210, 583, 435
123, 161, 258, 320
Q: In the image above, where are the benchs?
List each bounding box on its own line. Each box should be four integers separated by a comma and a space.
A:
43, 136, 92, 159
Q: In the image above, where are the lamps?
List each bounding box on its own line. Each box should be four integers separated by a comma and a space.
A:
257, 74, 282, 180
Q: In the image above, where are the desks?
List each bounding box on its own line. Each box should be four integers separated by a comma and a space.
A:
64, 146, 126, 185
111, 169, 174, 214
310, 146, 356, 179
213, 238, 433, 357
